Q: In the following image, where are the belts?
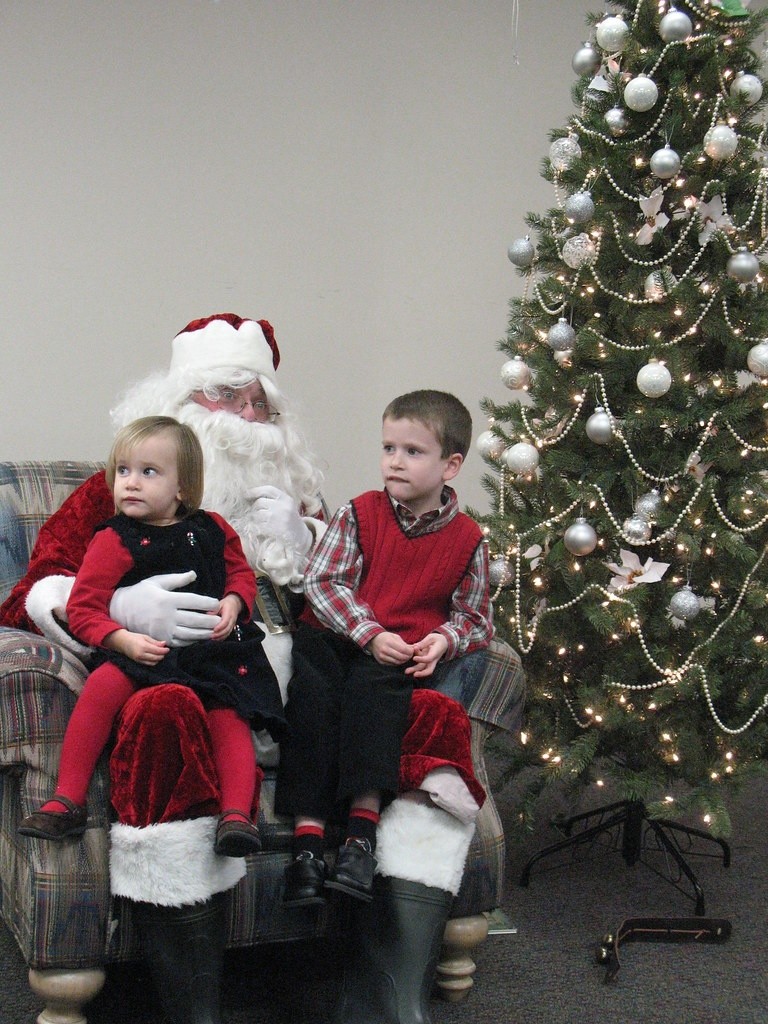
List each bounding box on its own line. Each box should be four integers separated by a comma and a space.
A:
253, 572, 309, 633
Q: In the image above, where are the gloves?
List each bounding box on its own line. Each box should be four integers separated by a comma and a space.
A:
108, 571, 222, 648
244, 485, 314, 557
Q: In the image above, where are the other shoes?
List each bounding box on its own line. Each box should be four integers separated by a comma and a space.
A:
214, 809, 263, 857
17, 795, 88, 839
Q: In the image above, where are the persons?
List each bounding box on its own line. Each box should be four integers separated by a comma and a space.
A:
19, 415, 263, 859
0, 313, 487, 1024
274, 389, 494, 908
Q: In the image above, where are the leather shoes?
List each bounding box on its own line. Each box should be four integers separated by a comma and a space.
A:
278, 860, 325, 907
323, 839, 379, 902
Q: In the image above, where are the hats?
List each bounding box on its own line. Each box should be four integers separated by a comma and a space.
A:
169, 313, 280, 386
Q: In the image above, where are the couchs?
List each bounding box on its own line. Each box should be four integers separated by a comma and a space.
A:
0, 460, 527, 1024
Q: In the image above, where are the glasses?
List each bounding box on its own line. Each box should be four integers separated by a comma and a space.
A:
193, 388, 281, 423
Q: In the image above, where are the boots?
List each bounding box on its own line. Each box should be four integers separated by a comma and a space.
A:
111, 813, 248, 1024
331, 802, 478, 1024
